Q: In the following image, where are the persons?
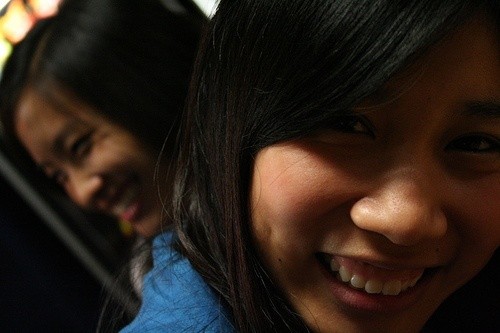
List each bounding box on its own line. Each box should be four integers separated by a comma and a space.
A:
119, 0, 500, 333
0, 0, 211, 242
